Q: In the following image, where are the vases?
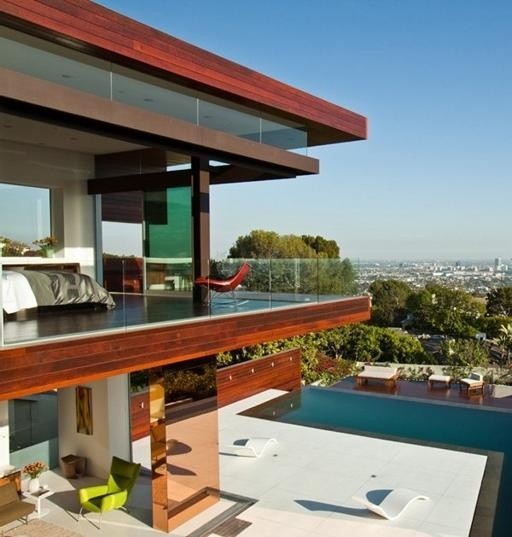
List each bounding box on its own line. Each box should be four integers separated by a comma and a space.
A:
26, 480, 41, 492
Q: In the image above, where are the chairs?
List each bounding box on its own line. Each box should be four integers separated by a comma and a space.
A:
227, 436, 279, 460
460, 372, 485, 396
194, 261, 252, 306
78, 455, 142, 528
352, 485, 430, 520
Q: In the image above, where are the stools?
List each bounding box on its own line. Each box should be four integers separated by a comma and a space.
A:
429, 374, 451, 389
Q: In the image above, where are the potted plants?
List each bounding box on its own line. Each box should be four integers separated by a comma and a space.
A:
34, 236, 58, 257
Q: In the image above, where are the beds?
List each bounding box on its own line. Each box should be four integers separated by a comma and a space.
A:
2, 263, 115, 319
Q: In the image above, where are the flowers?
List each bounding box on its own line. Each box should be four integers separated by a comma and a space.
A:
22, 461, 48, 478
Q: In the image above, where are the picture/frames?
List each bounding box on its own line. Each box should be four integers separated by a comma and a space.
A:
74, 386, 93, 437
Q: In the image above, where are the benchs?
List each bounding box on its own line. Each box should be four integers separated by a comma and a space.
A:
356, 365, 403, 388
0, 477, 36, 533
